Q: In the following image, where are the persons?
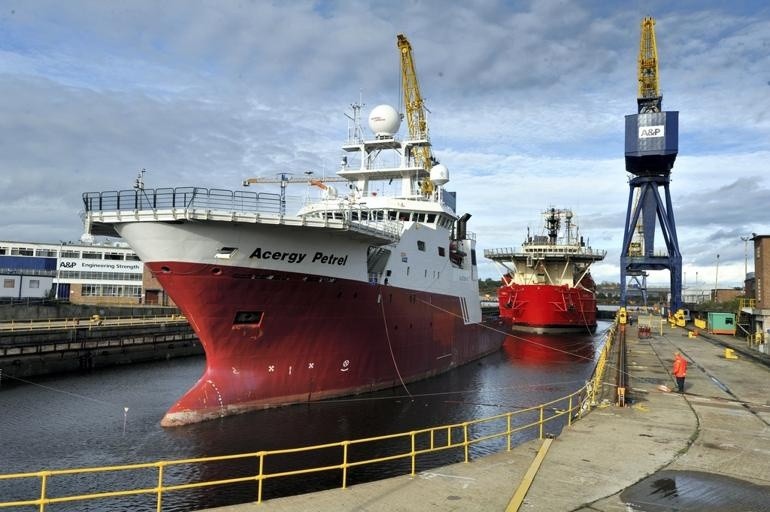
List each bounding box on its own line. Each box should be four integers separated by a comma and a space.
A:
672, 350, 688, 394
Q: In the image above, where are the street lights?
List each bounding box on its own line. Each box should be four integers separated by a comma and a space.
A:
741, 235, 750, 274
695, 270, 698, 304
714, 252, 721, 302
683, 271, 688, 302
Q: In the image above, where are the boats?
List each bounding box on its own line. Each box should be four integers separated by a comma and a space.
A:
78, 87, 515, 430
482, 204, 612, 336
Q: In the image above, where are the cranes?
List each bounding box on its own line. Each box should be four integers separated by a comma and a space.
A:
238, 170, 354, 216
